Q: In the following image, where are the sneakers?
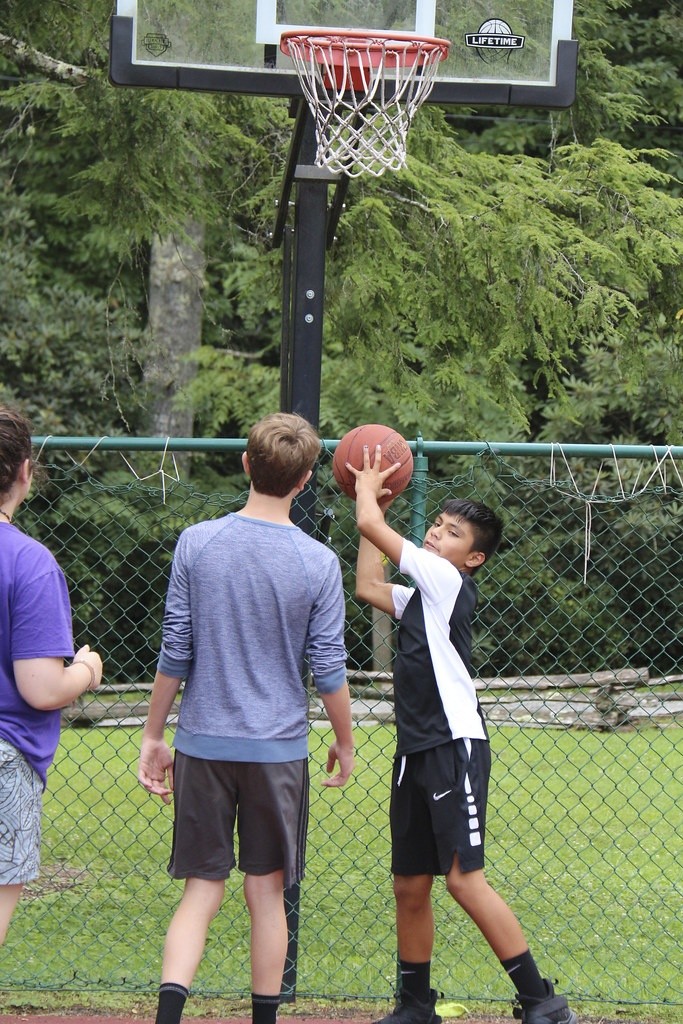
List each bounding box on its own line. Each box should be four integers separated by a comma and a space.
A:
513, 979, 578, 1024
369, 988, 442, 1024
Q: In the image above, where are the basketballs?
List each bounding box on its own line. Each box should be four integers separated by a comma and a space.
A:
331, 424, 413, 506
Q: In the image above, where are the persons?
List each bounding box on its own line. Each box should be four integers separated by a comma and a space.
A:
138, 414, 353, 1023
344, 445, 576, 1024
1, 400, 103, 951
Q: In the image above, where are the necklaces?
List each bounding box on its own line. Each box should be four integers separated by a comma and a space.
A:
0, 509, 12, 525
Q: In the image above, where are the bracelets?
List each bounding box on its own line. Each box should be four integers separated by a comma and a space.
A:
69, 660, 95, 690
